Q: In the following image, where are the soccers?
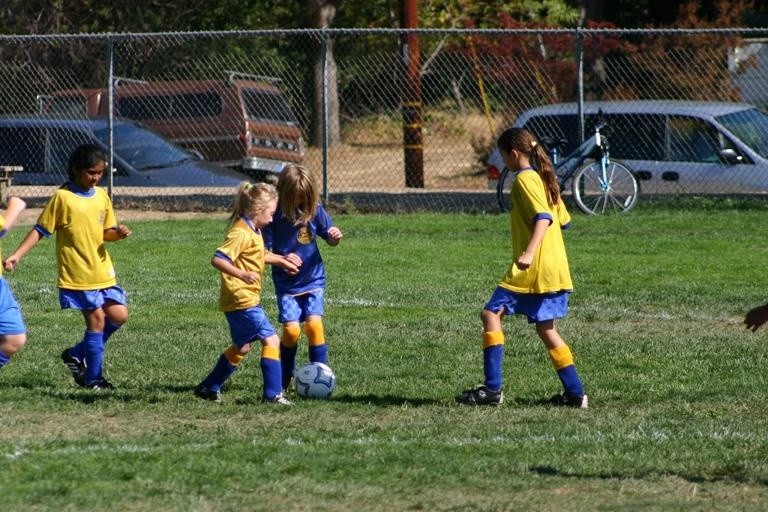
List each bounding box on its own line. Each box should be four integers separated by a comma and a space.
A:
294, 361, 335, 398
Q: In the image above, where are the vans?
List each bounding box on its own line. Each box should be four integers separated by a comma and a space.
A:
38, 70, 306, 178
486, 98, 767, 197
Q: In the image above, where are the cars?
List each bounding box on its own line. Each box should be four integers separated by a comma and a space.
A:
0, 115, 256, 188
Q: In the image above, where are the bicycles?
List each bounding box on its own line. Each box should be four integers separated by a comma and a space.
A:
496, 120, 641, 216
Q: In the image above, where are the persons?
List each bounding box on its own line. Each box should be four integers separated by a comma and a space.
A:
3, 142, 132, 392
742, 302, 767, 332
255, 162, 343, 390
190, 179, 294, 407
451, 125, 590, 411
0, 196, 29, 368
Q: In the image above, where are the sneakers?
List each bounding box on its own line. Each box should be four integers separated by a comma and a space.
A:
454, 383, 505, 407
261, 390, 295, 405
81, 373, 115, 391
60, 346, 86, 386
193, 380, 228, 405
546, 392, 589, 409
